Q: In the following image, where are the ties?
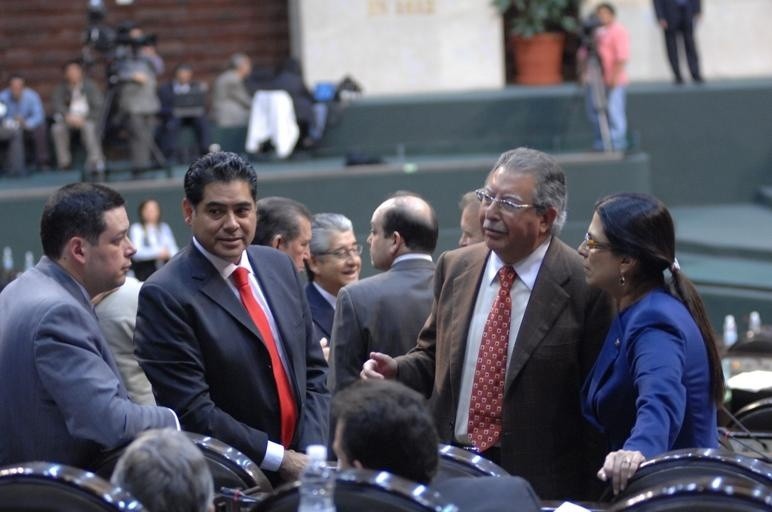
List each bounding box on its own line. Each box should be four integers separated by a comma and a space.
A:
467, 264, 519, 453
233, 269, 301, 454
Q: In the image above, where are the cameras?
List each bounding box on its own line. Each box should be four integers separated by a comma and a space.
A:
84, 3, 160, 52
556, 13, 605, 53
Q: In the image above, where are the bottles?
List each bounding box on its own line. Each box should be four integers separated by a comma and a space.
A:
299, 445, 334, 512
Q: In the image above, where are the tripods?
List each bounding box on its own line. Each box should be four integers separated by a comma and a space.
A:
81, 54, 173, 182
562, 56, 611, 152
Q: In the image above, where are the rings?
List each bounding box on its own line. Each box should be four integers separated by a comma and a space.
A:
621, 455, 631, 463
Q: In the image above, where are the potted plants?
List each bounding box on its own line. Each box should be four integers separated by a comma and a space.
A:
494, 1, 586, 89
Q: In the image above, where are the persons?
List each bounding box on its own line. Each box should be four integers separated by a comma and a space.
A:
127, 196, 181, 280
326, 188, 442, 387
0, 179, 185, 475
576, 189, 727, 494
0, 72, 52, 177
256, 192, 315, 287
107, 41, 161, 180
311, 210, 366, 361
652, 0, 707, 86
456, 185, 487, 253
358, 143, 613, 501
206, 53, 251, 138
575, 4, 633, 160
129, 146, 332, 488
326, 376, 547, 508
158, 64, 206, 166
241, 53, 320, 163
106, 424, 222, 512
45, 58, 114, 175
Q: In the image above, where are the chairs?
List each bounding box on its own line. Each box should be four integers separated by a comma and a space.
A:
247, 468, 439, 512
96, 432, 268, 502
727, 337, 771, 352
0, 461, 141, 511
430, 445, 512, 487
597, 448, 769, 497
728, 399, 771, 433
610, 478, 770, 511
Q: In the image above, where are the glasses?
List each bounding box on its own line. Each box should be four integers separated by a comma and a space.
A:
313, 244, 364, 257
583, 232, 615, 254
474, 190, 538, 213
